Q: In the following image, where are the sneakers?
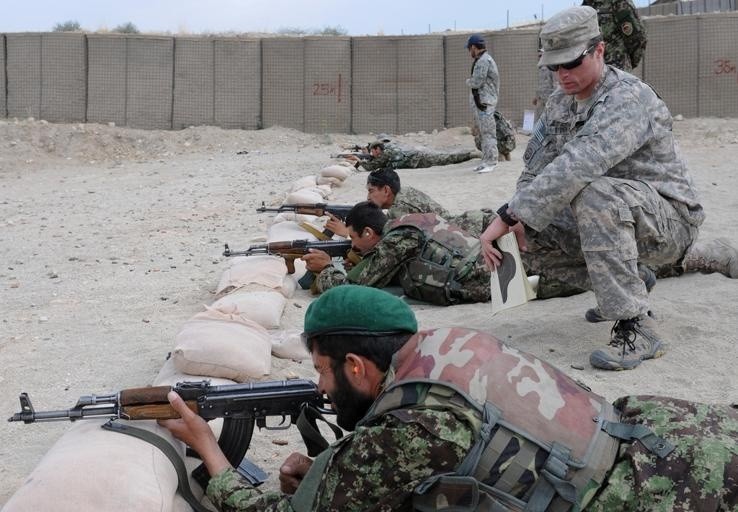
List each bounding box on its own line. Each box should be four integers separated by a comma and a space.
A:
590, 311, 663, 369
474, 163, 498, 173
499, 153, 511, 161
585, 262, 656, 322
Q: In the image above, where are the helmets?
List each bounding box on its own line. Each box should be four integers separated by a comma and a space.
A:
377, 134, 390, 142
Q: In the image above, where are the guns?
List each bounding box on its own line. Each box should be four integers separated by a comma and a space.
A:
7, 376, 337, 495
257, 201, 354, 240
331, 154, 376, 170
343, 143, 371, 153
223, 239, 360, 290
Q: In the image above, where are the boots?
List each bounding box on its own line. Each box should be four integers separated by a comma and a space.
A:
470, 150, 483, 158
682, 236, 738, 278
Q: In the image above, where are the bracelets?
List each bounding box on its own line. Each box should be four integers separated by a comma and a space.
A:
496, 202, 518, 228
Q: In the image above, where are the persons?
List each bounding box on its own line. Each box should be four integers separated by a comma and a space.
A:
345, 141, 483, 172
463, 33, 500, 173
360, 133, 401, 154
324, 166, 497, 240
480, 4, 705, 372
470, 109, 519, 162
155, 283, 738, 512
299, 199, 738, 311
581, 0, 650, 75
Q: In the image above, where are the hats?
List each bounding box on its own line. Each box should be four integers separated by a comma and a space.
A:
464, 34, 484, 48
369, 142, 382, 149
538, 6, 599, 69
304, 284, 417, 339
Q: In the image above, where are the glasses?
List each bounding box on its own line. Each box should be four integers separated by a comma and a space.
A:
547, 45, 595, 71
